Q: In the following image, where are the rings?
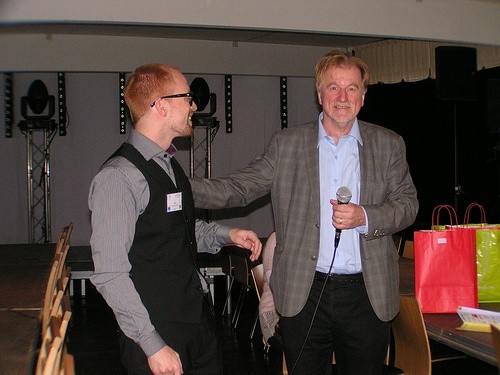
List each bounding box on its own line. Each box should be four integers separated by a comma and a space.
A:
340, 219, 343, 224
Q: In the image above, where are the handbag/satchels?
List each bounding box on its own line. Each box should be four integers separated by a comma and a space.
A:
432, 202, 500, 302
414, 205, 478, 313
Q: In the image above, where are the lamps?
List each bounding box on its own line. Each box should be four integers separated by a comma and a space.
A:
20, 79, 56, 120
189, 77, 217, 117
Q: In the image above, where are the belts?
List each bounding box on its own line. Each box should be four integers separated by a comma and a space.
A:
315, 272, 362, 283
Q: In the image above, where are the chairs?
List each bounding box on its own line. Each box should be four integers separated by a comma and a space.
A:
392, 296, 432, 375
221, 255, 289, 375
0, 221, 78, 375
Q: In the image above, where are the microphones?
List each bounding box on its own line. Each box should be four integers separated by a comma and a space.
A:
334, 186, 352, 248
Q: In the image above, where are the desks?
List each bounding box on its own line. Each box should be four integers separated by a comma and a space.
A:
66, 259, 223, 306
398, 257, 500, 375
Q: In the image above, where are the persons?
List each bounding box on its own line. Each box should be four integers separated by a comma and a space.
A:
187, 51, 419, 375
259, 231, 282, 348
87, 64, 262, 375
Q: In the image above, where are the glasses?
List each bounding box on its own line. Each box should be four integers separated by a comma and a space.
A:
150, 92, 193, 107
